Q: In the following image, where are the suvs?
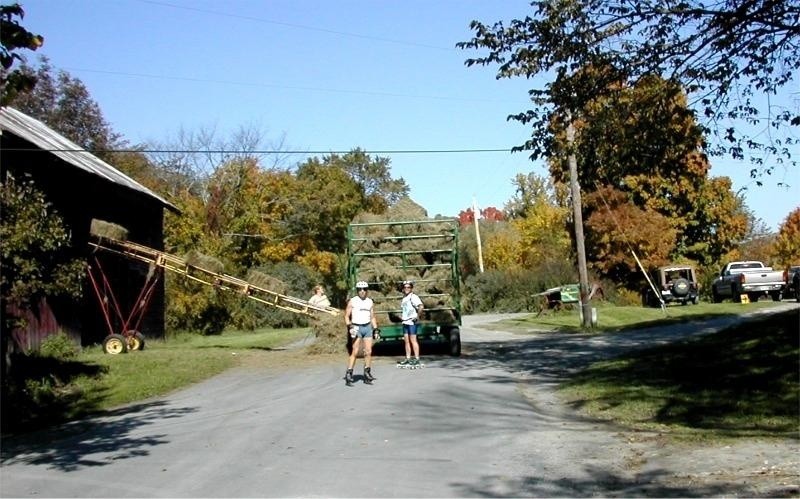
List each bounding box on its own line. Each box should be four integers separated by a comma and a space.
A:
645, 265, 699, 306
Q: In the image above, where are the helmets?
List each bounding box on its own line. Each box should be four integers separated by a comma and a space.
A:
403, 281, 413, 285
356, 281, 368, 287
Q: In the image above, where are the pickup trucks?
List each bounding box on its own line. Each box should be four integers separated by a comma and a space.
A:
710, 260, 788, 302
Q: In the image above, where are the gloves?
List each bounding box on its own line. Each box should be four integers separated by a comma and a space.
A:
373, 328, 380, 340
348, 324, 356, 338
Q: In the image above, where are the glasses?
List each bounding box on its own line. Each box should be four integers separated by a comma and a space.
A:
403, 287, 412, 289
357, 288, 368, 290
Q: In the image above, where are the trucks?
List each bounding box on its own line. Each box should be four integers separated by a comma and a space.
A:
346, 219, 461, 358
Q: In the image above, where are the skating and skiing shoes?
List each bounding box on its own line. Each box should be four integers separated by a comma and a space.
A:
397, 359, 425, 370
345, 369, 354, 388
363, 367, 377, 384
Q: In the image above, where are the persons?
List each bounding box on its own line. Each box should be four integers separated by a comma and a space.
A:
342, 281, 381, 382
395, 281, 424, 365
307, 286, 331, 308
